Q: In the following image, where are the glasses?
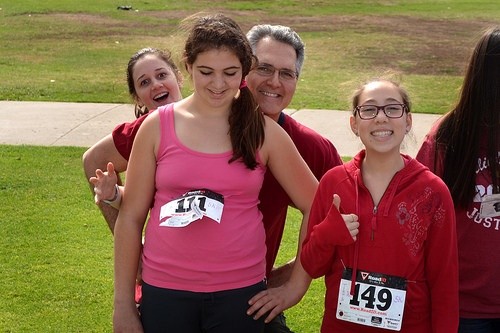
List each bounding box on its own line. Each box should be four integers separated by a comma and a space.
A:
356, 103, 408, 120
253, 64, 298, 81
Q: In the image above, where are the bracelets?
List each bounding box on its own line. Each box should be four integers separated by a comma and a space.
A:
107, 183, 119, 201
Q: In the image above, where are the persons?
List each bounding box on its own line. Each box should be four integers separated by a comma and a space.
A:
300, 79, 459, 332
84, 25, 344, 288
89, 46, 184, 212
113, 15, 319, 333
415, 26, 500, 332
